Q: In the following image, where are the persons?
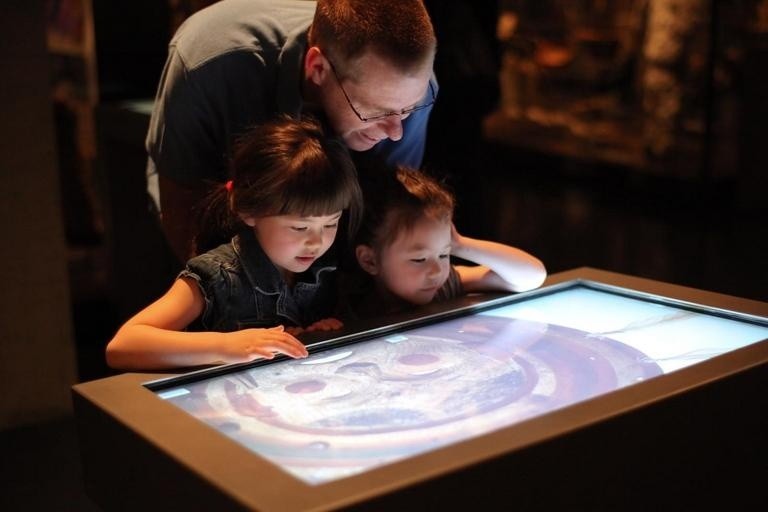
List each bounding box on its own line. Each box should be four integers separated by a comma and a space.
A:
106, 118, 547, 371
423, 0, 549, 241
145, 2, 441, 269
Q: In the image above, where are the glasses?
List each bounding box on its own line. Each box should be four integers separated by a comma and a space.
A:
325, 56, 435, 122
